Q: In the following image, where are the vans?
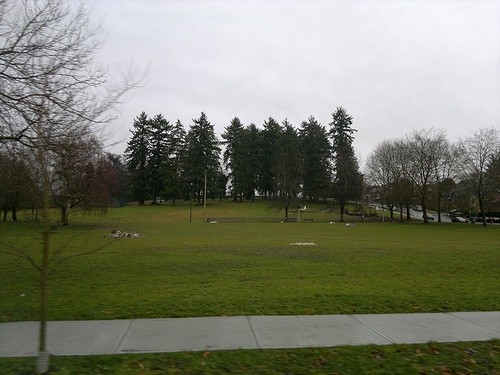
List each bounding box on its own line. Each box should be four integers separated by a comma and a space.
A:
412, 205, 423, 211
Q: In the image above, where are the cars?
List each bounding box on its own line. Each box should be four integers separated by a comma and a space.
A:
423, 214, 434, 219
452, 216, 469, 224
